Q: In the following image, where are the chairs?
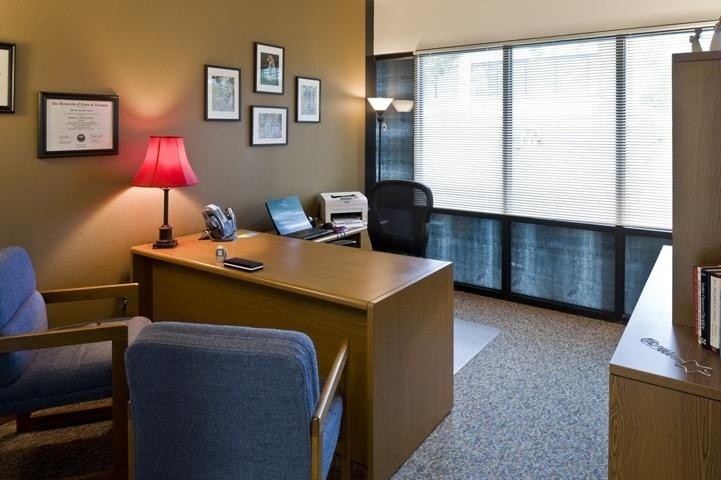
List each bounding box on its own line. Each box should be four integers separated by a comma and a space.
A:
367, 180, 434, 259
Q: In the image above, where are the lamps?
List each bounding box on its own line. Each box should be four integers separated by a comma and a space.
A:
365, 96, 395, 184
392, 99, 415, 113
130, 135, 200, 249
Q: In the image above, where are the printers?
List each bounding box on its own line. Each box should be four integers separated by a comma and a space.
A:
320, 192, 368, 228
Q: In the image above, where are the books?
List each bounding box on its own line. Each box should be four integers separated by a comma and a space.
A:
697, 266, 721, 344
700, 269, 721, 349
710, 273, 721, 350
693, 265, 699, 337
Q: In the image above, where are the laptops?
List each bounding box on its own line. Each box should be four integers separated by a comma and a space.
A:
264, 196, 334, 240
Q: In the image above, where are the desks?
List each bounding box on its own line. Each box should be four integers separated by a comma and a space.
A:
128, 228, 454, 479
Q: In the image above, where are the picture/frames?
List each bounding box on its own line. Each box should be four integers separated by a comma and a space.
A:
204, 41, 322, 147
0, 42, 15, 114
37, 90, 120, 159
